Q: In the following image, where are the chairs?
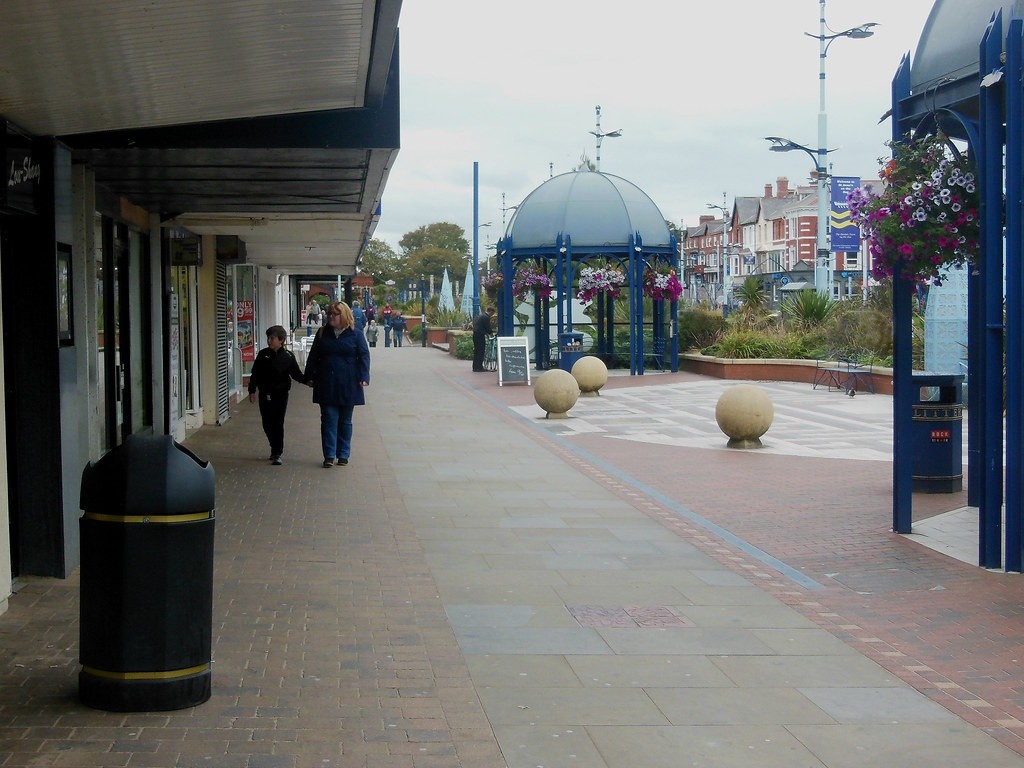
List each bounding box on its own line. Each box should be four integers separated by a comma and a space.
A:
289, 335, 316, 366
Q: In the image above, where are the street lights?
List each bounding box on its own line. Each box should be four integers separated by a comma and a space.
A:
498, 192, 520, 231
761, 0, 881, 311
473, 221, 493, 326
705, 192, 728, 317
588, 105, 624, 171
484, 234, 498, 279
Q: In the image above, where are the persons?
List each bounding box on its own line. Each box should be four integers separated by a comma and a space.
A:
382, 303, 393, 318
305, 300, 328, 327
365, 320, 379, 347
471, 307, 495, 371
305, 301, 370, 468
350, 301, 366, 334
389, 311, 408, 347
248, 324, 304, 465
365, 303, 376, 326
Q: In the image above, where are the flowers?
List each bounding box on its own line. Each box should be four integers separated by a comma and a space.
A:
480, 272, 504, 298
578, 260, 627, 303
643, 263, 683, 302
846, 131, 1007, 294
512, 268, 552, 301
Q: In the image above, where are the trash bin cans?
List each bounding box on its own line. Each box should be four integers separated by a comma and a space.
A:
79, 438, 221, 713
558, 333, 585, 373
889, 374, 964, 493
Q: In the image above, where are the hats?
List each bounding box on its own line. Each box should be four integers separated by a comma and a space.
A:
311, 300, 316, 303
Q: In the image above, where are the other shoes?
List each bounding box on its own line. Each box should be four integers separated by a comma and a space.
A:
273, 455, 282, 465
473, 368, 488, 372
337, 459, 348, 465
324, 459, 334, 467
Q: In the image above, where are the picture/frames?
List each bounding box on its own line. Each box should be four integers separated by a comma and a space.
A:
56, 241, 75, 348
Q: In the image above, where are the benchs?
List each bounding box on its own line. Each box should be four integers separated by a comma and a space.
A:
812, 350, 878, 396
600, 334, 666, 372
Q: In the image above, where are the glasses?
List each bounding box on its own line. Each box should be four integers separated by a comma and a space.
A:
326, 312, 340, 316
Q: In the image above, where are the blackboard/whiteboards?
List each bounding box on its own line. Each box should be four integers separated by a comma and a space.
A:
498, 345, 530, 382
